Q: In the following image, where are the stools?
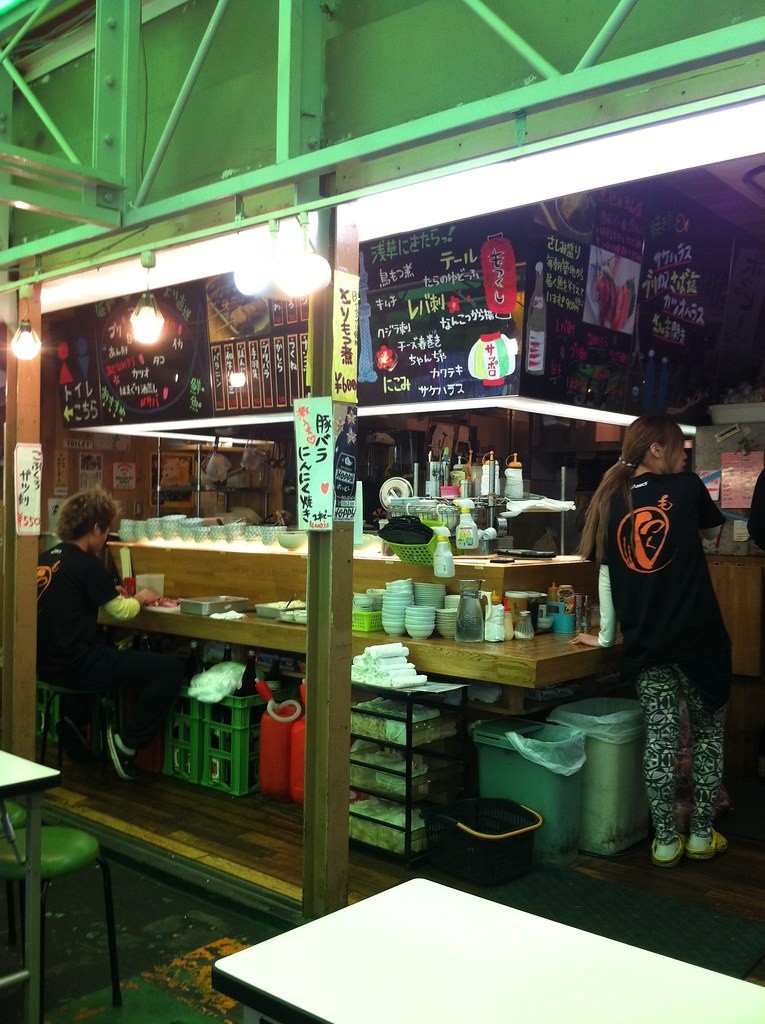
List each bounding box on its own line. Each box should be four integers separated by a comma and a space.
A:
0, 802, 121, 1024
38, 682, 108, 764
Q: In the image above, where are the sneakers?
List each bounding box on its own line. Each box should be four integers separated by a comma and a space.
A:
55, 716, 95, 768
106, 724, 138, 780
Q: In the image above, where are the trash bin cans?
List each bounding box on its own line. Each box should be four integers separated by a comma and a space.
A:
471, 720, 588, 860
547, 697, 651, 854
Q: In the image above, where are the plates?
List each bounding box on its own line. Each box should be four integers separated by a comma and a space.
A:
207, 294, 269, 336
586, 264, 630, 334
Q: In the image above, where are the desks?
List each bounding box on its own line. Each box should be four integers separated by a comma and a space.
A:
0, 749, 61, 1024
211, 877, 765, 1024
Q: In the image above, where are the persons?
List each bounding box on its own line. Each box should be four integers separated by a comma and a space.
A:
37, 490, 161, 780
748, 467, 765, 558
566, 414, 733, 865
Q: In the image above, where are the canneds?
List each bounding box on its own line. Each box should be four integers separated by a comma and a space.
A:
576, 593, 591, 633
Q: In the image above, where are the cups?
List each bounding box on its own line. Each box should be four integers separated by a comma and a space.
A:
135, 573, 165, 597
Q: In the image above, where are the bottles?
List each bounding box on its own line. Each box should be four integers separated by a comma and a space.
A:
484, 582, 600, 643
131, 629, 282, 784
424, 447, 524, 500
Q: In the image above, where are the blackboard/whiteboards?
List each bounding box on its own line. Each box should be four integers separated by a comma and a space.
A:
354, 217, 528, 397
52, 271, 312, 430
524, 188, 765, 422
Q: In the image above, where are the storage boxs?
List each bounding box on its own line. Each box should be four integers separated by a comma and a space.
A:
37, 688, 117, 760
160, 638, 300, 797
707, 402, 765, 425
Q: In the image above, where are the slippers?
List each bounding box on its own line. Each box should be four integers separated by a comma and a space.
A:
651, 832, 685, 867
683, 827, 728, 859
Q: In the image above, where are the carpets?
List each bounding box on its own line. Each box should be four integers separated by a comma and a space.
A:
481, 868, 765, 977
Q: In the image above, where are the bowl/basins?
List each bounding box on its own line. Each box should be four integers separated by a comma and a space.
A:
280, 609, 307, 623
352, 578, 460, 640
117, 514, 308, 553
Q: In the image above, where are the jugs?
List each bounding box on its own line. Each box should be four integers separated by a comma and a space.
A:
454, 579, 493, 643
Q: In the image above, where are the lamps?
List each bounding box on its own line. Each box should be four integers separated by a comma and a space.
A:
130, 251, 164, 344
10, 284, 42, 361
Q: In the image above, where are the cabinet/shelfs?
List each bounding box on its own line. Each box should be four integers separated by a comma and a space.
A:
350, 683, 469, 871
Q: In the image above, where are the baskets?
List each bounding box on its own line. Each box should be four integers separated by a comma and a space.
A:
383, 520, 452, 567
352, 611, 384, 632
418, 799, 543, 886
